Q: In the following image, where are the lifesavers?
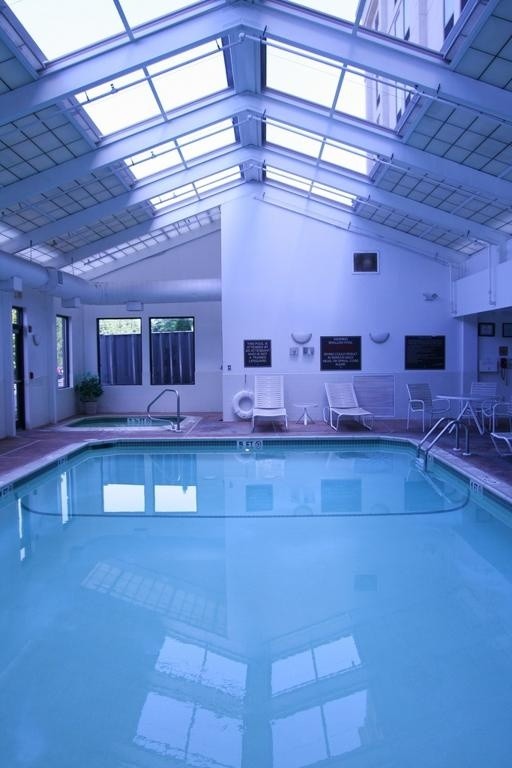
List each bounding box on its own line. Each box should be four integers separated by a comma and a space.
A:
233, 391, 254, 419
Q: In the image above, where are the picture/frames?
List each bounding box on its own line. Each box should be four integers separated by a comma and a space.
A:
478, 322, 496, 337
502, 322, 511, 337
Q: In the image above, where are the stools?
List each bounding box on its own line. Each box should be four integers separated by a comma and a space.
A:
480, 398, 511, 457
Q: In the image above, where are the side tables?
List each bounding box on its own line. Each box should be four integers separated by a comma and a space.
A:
293, 402, 318, 426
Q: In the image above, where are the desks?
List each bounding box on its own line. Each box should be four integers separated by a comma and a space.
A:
436, 394, 497, 436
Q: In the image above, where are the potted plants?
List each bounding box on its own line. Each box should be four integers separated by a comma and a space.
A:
74, 369, 105, 415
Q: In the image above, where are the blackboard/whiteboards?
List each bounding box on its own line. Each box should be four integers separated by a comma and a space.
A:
405, 335, 446, 370
320, 336, 362, 371
244, 340, 271, 367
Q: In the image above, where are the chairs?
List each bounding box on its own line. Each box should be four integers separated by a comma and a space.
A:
466, 381, 498, 433
250, 374, 288, 434
322, 382, 375, 431
404, 382, 450, 433
